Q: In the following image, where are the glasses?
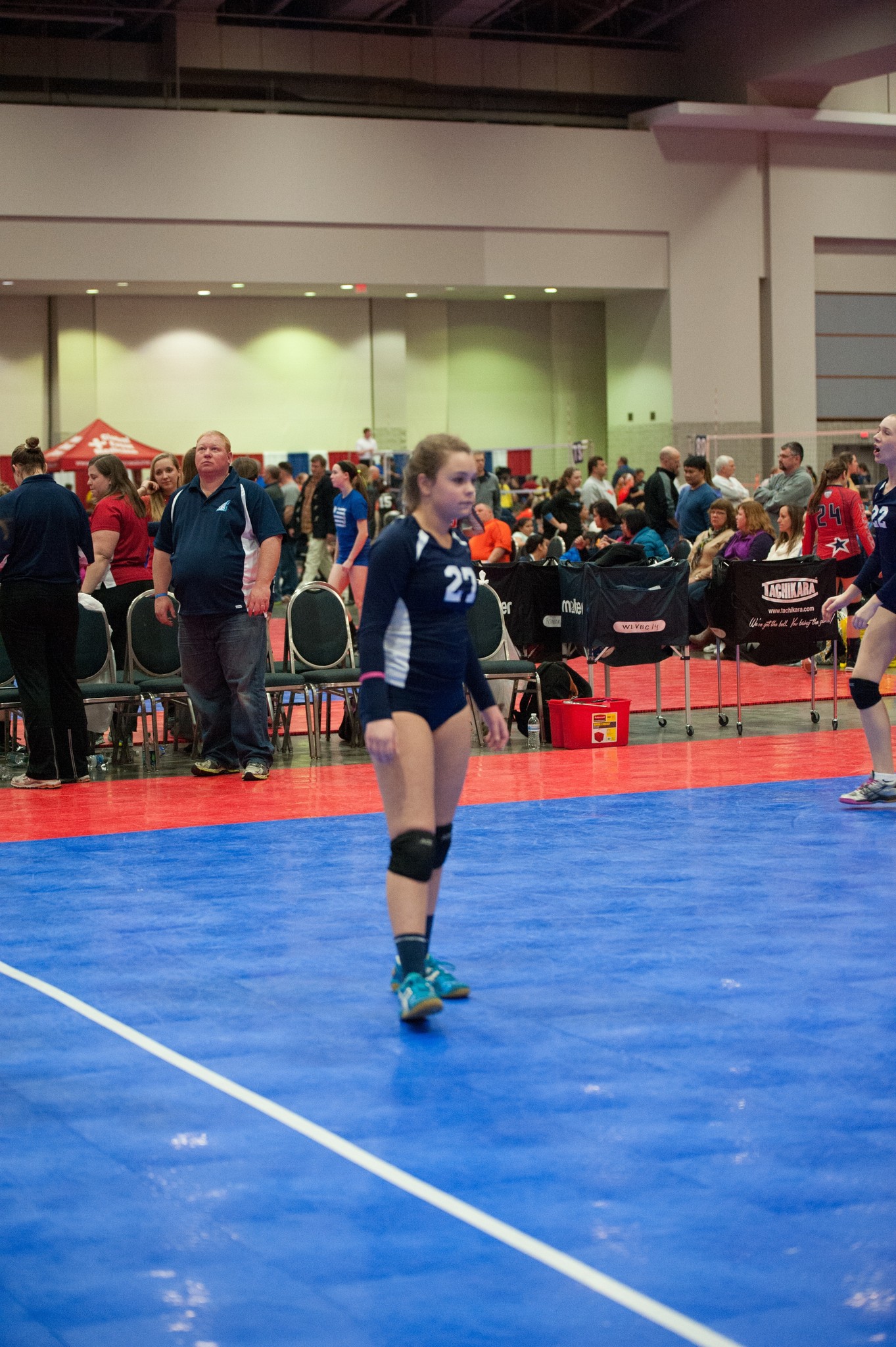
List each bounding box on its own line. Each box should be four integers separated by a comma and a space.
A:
777, 454, 797, 459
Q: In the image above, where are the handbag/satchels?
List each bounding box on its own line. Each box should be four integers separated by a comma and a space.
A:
337, 696, 359, 744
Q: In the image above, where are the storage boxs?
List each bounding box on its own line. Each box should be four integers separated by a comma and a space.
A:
546, 697, 631, 749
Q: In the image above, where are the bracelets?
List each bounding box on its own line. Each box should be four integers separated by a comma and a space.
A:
155, 592, 167, 599
358, 671, 384, 683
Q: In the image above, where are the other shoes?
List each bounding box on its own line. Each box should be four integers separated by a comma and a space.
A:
801, 655, 817, 676
845, 660, 855, 672
107, 731, 133, 746
90, 734, 105, 746
168, 716, 175, 730
0, 736, 28, 754
346, 625, 360, 657
703, 641, 726, 655
689, 625, 716, 644
169, 728, 185, 739
267, 712, 283, 728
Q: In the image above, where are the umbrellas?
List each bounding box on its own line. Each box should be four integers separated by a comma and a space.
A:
43, 417, 181, 468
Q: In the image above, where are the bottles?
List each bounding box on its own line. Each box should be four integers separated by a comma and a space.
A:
157, 746, 166, 757
101, 747, 140, 758
85, 753, 108, 768
142, 732, 156, 771
0, 751, 27, 765
527, 713, 540, 750
0, 763, 16, 781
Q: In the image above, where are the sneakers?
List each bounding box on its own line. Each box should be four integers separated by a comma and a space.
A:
61, 774, 91, 783
242, 762, 270, 779
390, 953, 471, 998
397, 971, 444, 1020
10, 772, 62, 789
191, 759, 241, 777
839, 770, 896, 804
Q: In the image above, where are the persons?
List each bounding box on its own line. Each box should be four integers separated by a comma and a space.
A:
822, 414, 896, 810
0, 428, 876, 789
351, 434, 510, 1019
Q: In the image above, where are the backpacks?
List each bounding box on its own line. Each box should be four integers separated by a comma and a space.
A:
588, 541, 670, 660
813, 610, 848, 665
511, 661, 593, 743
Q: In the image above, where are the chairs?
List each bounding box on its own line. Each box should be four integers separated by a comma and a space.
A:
263, 581, 364, 760
0, 633, 31, 767
462, 579, 546, 747
669, 539, 692, 563
546, 535, 566, 558
75, 589, 203, 773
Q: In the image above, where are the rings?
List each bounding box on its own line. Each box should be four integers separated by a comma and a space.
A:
260, 605, 265, 606
